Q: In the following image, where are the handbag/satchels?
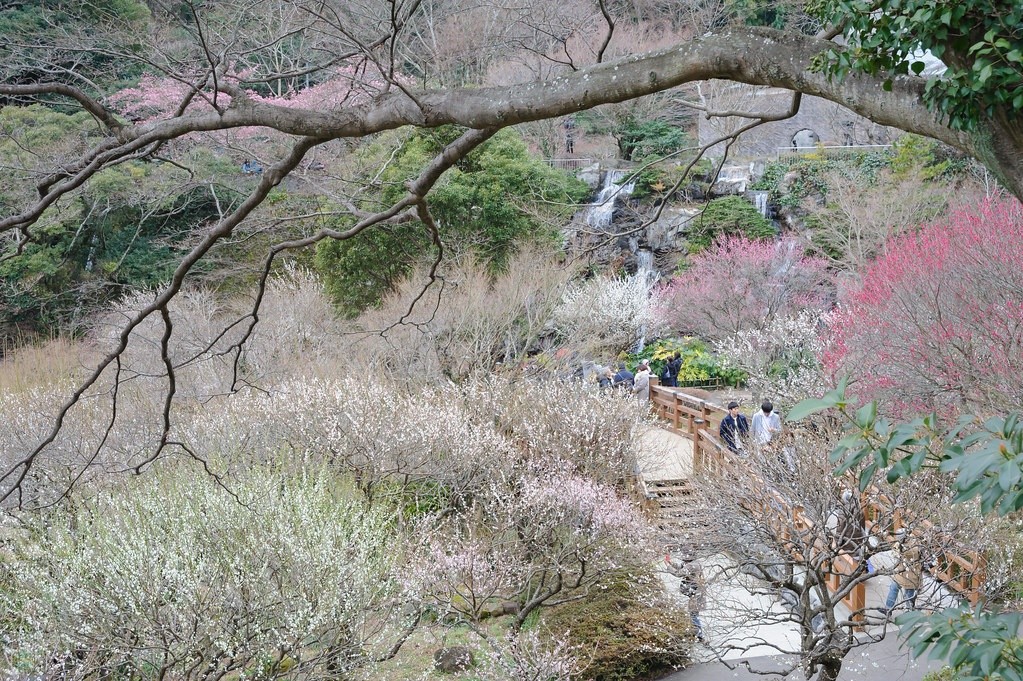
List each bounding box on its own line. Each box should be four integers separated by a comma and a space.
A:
622, 379, 633, 393
664, 366, 670, 378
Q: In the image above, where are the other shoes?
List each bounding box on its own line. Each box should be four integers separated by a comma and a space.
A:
877, 607, 892, 617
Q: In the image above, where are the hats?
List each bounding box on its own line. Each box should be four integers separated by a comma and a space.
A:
643, 359, 648, 366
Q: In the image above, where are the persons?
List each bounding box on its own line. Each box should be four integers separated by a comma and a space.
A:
584, 348, 684, 402
749, 402, 784, 451
876, 526, 923, 616
835, 489, 865, 559
719, 402, 747, 454
